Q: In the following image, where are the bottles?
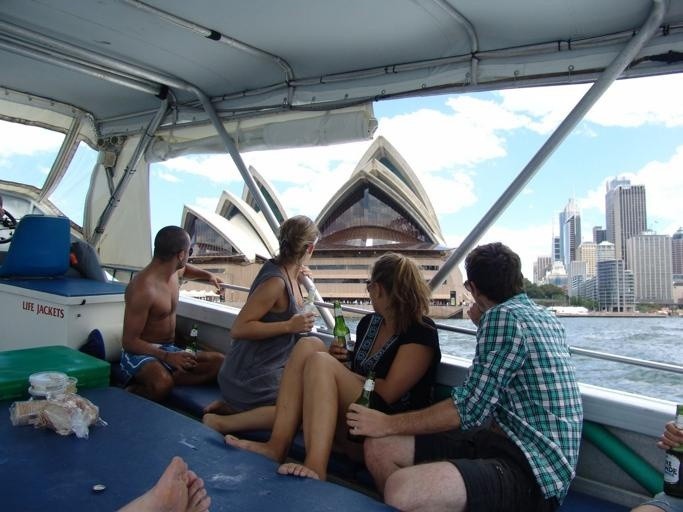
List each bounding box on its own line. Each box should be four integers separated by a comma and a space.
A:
332, 301, 353, 362
296, 287, 317, 337
182, 323, 199, 373
345, 370, 375, 443
663, 404, 683, 499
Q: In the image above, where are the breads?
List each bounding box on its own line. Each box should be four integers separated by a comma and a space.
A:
14, 397, 98, 433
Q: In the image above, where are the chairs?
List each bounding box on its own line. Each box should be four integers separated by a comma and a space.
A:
0, 215, 71, 279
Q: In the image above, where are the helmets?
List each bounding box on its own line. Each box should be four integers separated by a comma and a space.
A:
463, 280, 472, 293
367, 272, 384, 292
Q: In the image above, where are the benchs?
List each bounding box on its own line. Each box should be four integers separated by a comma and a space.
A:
88, 326, 629, 512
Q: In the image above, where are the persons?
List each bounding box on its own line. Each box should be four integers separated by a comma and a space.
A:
628, 417, 683, 511
344, 240, 585, 510
113, 224, 226, 405
221, 248, 442, 486
113, 454, 212, 512
199, 213, 322, 434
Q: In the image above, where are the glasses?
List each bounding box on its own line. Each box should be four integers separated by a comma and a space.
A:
189, 247, 193, 256
